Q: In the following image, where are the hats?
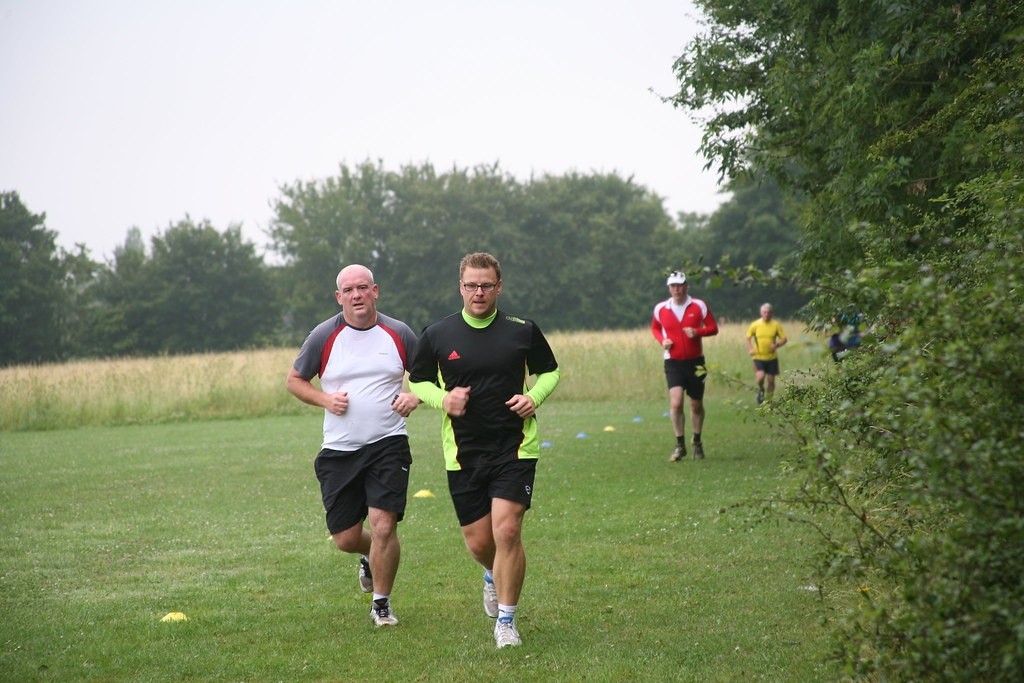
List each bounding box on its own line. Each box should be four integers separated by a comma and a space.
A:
667, 270, 687, 286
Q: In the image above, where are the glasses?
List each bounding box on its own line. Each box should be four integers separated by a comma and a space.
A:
461, 280, 499, 292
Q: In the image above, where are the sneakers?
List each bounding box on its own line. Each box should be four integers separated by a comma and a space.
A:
359, 554, 374, 592
691, 435, 705, 460
494, 616, 524, 650
483, 572, 500, 618
669, 445, 687, 462
369, 598, 399, 628
757, 388, 764, 403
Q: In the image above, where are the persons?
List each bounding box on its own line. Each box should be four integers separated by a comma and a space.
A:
408, 253, 561, 647
820, 307, 865, 363
746, 302, 787, 403
652, 272, 720, 461
286, 265, 438, 627
